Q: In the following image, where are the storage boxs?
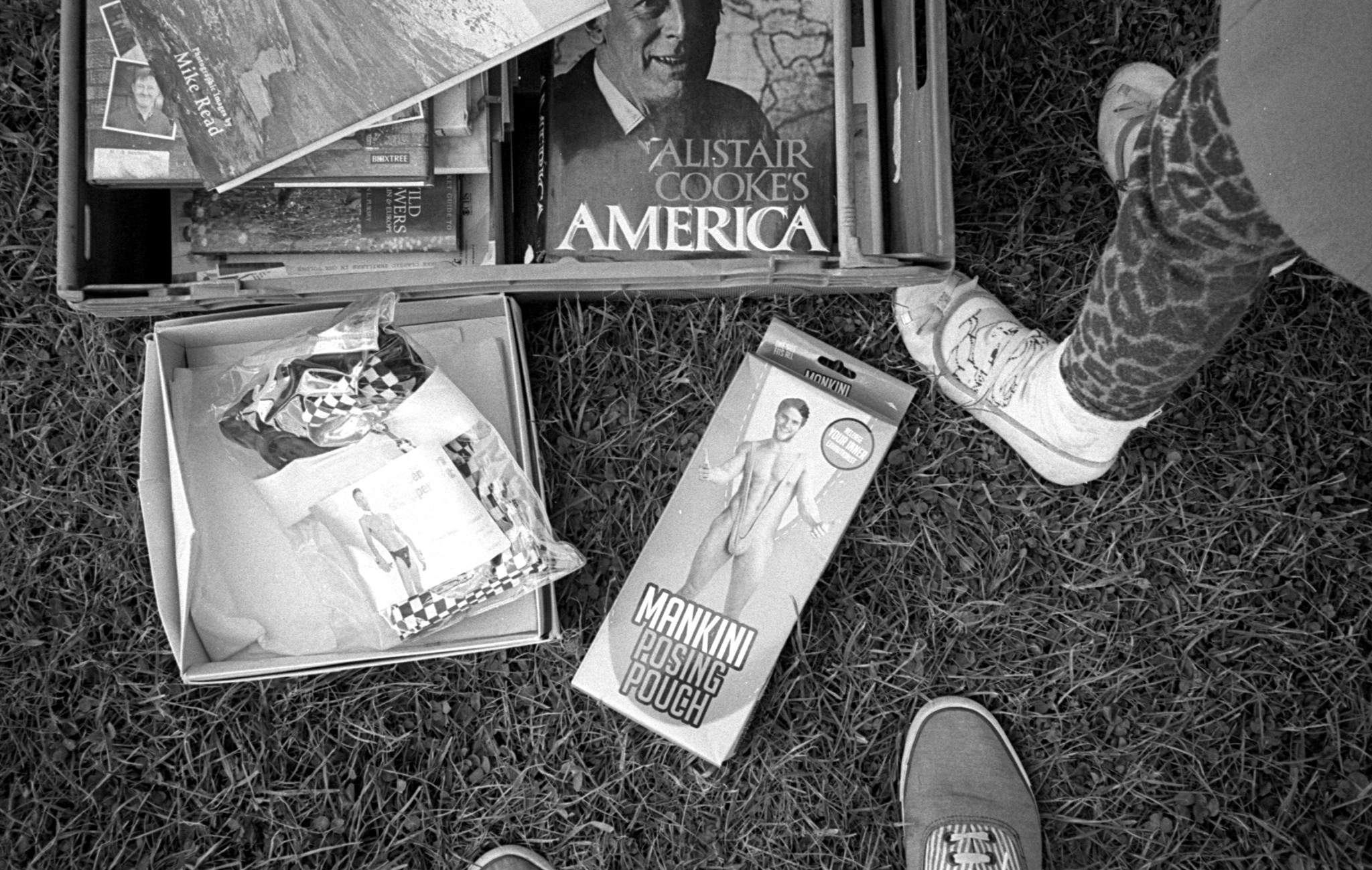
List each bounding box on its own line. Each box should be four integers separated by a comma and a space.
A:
569, 317, 919, 766
54, 0, 956, 320
134, 287, 563, 689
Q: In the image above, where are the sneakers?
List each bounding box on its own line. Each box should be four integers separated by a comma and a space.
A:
894, 695, 1049, 868
893, 265, 1163, 484
1095, 61, 1302, 275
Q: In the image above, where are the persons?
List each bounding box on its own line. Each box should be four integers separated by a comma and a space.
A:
553, 0, 798, 257
673, 397, 833, 625
476, 697, 1042, 870
109, 68, 173, 139
894, 0, 1372, 485
350, 486, 429, 601
